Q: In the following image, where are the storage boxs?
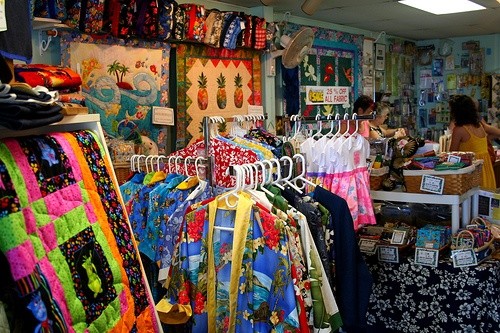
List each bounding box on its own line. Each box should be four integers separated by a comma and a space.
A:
414, 224, 446, 249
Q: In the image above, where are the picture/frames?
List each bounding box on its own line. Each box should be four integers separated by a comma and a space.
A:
404, 40, 415, 55
375, 43, 386, 71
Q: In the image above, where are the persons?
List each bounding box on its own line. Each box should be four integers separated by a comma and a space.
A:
450, 95, 500, 217
450, 98, 479, 135
339, 94, 374, 139
368, 101, 406, 139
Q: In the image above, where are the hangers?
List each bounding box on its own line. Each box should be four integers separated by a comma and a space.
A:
287, 113, 360, 145
212, 153, 316, 233
196, 113, 265, 146
130, 154, 207, 190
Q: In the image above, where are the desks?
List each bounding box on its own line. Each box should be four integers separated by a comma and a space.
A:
369, 187, 477, 235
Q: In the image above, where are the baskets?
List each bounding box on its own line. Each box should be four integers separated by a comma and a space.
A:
403, 158, 484, 195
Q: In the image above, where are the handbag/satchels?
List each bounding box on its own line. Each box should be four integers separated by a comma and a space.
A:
34, 0, 269, 50
487, 137, 497, 164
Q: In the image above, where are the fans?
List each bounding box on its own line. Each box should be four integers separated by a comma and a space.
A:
266, 25, 314, 77
